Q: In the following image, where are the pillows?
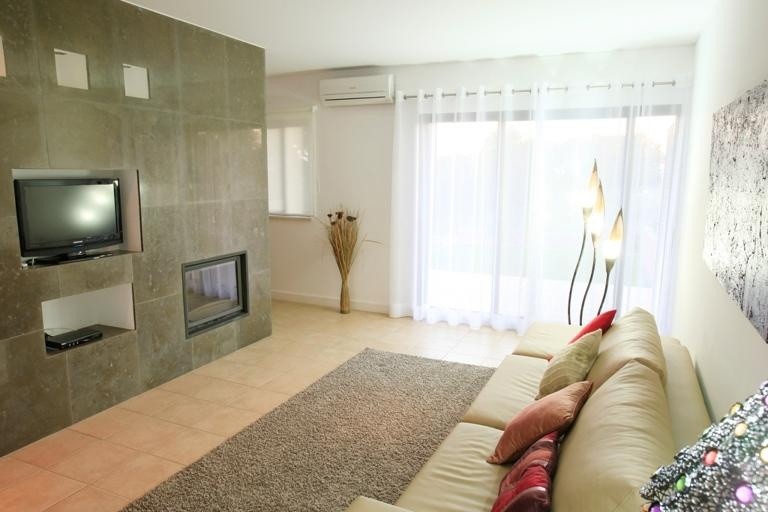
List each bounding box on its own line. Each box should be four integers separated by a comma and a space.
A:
551, 358, 676, 512
535, 327, 602, 400
488, 380, 591, 465
492, 430, 562, 512
569, 309, 618, 343
585, 306, 666, 393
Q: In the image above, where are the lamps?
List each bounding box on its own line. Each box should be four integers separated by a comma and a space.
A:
568, 157, 625, 325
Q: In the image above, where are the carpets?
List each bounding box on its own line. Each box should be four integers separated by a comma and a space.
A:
118, 347, 496, 512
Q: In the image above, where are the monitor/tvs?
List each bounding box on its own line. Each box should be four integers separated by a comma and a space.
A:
14, 178, 124, 264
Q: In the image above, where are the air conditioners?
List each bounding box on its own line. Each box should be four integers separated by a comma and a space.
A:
318, 73, 394, 108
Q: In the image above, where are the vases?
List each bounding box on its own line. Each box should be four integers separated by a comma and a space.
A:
340, 280, 350, 313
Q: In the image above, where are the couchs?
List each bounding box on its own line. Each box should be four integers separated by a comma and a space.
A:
346, 309, 714, 512
